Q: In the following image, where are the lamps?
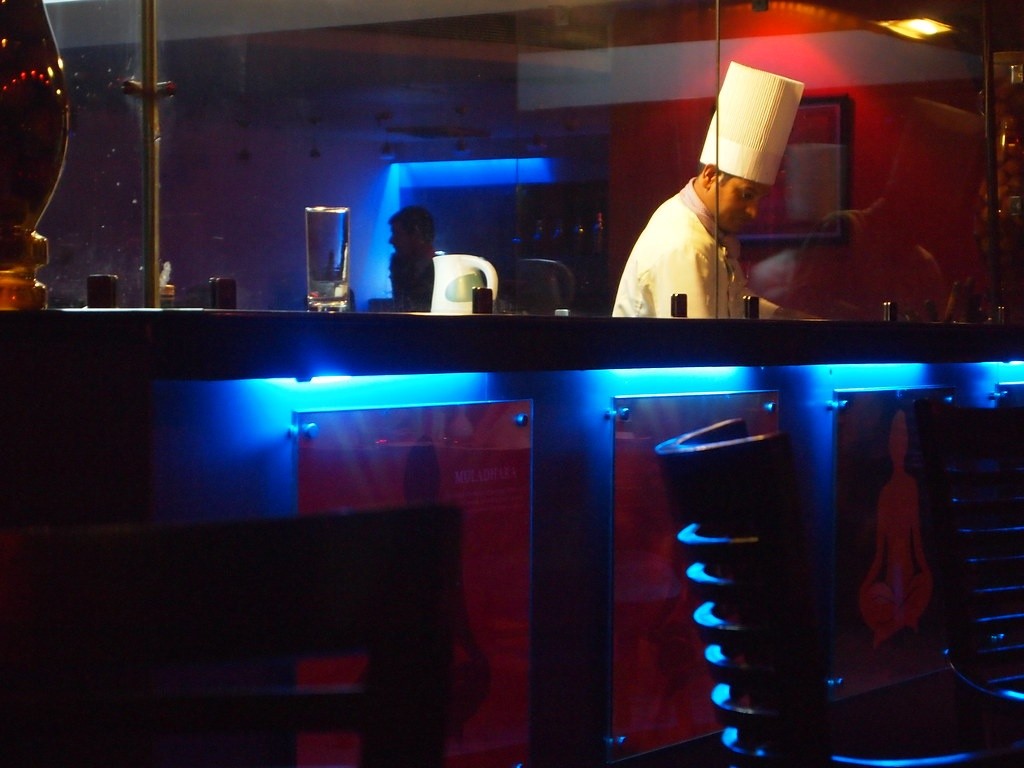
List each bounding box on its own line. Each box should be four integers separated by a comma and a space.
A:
237, 120, 252, 157
306, 116, 322, 156
374, 112, 396, 159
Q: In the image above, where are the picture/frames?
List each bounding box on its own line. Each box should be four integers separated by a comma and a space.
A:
737, 96, 851, 246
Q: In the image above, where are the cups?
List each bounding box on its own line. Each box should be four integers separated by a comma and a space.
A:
304, 206, 351, 312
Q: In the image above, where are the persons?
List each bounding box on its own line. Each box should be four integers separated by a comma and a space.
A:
389, 206, 445, 312
613, 63, 817, 319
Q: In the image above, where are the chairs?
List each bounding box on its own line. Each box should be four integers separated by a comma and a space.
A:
655, 418, 1024, 768
913, 399, 1024, 750
0, 494, 464, 768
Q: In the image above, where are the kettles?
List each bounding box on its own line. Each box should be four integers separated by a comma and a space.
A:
431, 251, 499, 315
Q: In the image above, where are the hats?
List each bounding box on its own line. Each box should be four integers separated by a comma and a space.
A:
700, 61, 804, 186
